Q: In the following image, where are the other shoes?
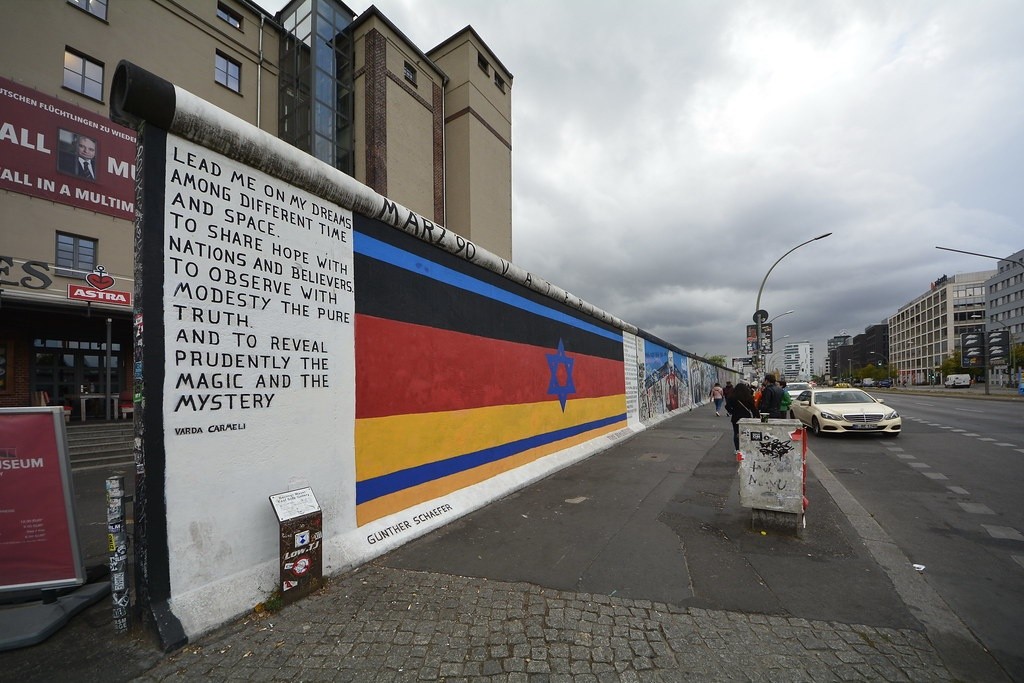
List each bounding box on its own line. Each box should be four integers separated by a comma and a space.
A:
716, 412, 720, 417
726, 412, 730, 417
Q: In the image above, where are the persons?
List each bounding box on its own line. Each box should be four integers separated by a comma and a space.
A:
709, 372, 791, 455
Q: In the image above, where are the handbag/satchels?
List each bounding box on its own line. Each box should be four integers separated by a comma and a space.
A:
749, 410, 753, 417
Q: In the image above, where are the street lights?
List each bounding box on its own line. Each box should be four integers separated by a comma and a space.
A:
870, 352, 890, 380
971, 314, 1016, 388
752, 231, 834, 383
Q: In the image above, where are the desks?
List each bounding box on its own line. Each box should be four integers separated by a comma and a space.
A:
80, 393, 120, 422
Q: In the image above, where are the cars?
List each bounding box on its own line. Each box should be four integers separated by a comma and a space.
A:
787, 387, 902, 438
876, 380, 891, 388
783, 382, 813, 406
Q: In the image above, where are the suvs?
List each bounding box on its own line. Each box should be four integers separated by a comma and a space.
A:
852, 377, 874, 387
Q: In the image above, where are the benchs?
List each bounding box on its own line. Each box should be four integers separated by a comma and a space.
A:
119, 404, 134, 420
41, 391, 72, 422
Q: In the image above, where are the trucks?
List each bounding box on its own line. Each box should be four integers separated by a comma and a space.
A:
944, 374, 972, 388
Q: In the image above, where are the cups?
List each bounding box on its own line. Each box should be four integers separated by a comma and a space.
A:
760, 413, 769, 423
